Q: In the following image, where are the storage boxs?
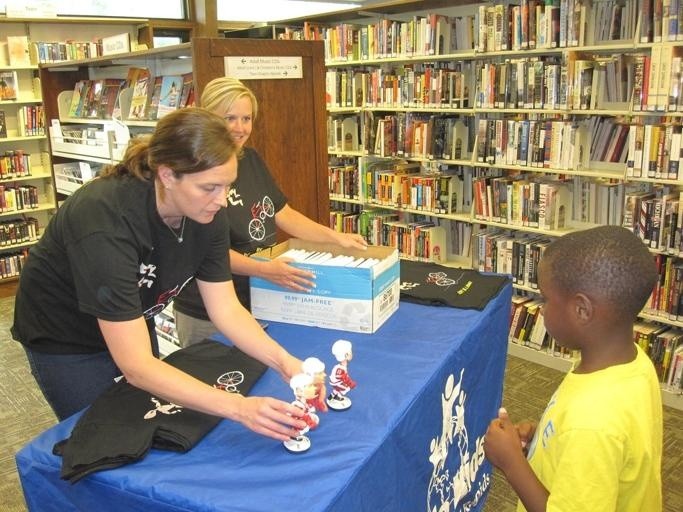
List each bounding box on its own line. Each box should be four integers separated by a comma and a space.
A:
247, 237, 400, 339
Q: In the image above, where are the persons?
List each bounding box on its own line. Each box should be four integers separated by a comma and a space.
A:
484, 223, 666, 512
9, 108, 330, 442
174, 77, 367, 348
282, 340, 356, 452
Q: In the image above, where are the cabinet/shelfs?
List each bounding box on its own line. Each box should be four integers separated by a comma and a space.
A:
272, 3, 682, 415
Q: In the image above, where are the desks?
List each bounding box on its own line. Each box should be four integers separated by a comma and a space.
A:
15, 272, 515, 511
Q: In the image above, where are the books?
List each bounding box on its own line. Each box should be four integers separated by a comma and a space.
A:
268, 1, 682, 397
0, 15, 195, 358
274, 247, 382, 273
